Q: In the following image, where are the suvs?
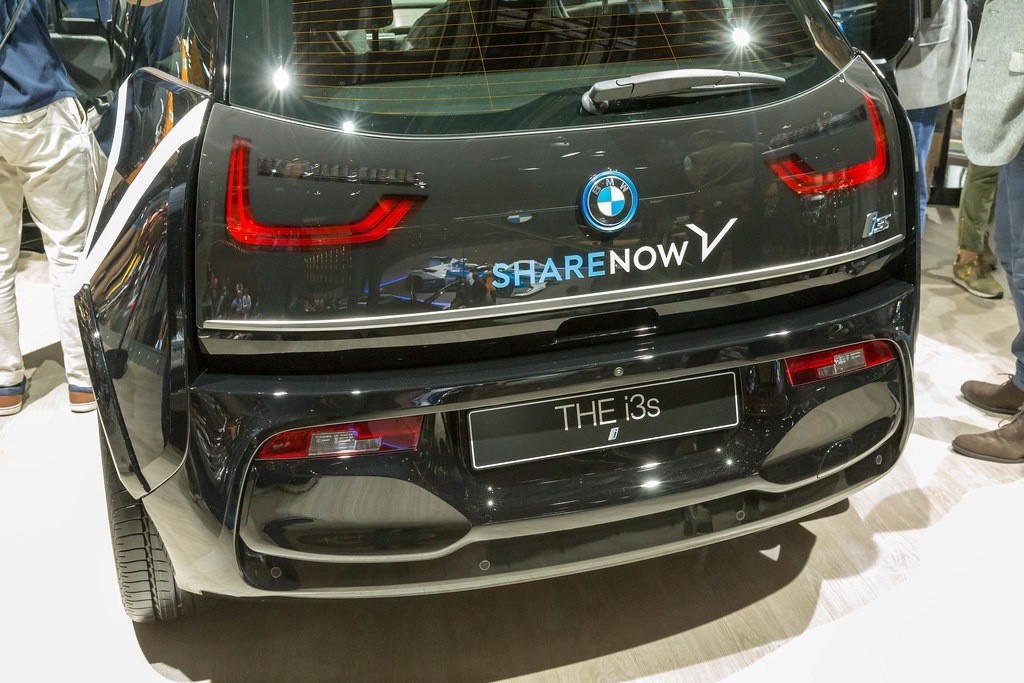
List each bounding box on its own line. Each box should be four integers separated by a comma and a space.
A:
47, 2, 925, 630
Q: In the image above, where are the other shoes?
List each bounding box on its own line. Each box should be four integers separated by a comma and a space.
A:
69, 388, 97, 413
0, 375, 27, 415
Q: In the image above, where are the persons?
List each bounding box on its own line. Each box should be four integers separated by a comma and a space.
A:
202, 262, 251, 320
256, 161, 305, 320
684, 129, 839, 276
450, 264, 497, 309
96, 0, 188, 205
952, 161, 1004, 298
952, 0, 1024, 463
893, 0, 973, 236
0, 0, 163, 416
348, 199, 386, 314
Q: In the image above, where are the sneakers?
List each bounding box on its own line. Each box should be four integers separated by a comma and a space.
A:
953, 253, 1005, 300
983, 232, 998, 267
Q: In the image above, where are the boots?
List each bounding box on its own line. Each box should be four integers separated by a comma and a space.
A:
952, 402, 1024, 464
960, 372, 1024, 416
